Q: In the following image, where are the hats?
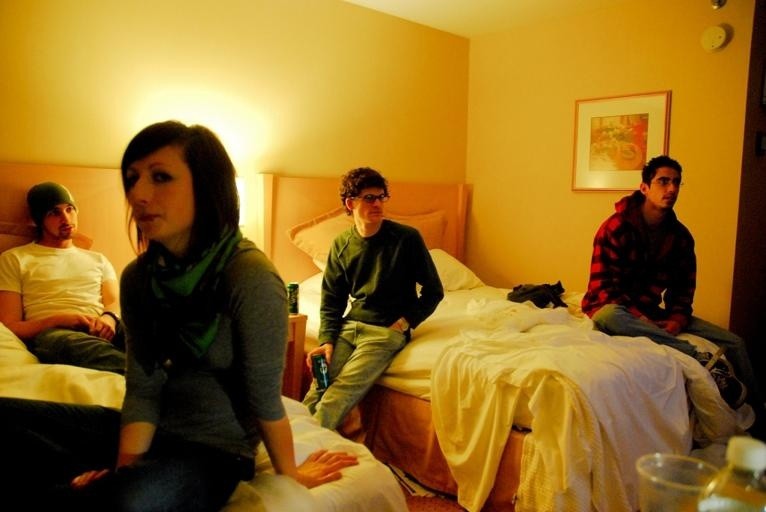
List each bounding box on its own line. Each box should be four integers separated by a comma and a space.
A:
25, 181, 79, 225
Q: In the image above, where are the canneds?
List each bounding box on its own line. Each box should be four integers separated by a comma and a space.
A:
312, 353, 330, 392
287, 282, 300, 314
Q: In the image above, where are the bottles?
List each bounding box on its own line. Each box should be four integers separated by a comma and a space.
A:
699, 435, 764, 511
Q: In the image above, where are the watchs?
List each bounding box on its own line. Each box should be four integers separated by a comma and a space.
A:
397, 318, 409, 332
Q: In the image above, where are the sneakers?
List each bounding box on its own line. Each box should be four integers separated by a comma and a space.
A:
700, 351, 748, 410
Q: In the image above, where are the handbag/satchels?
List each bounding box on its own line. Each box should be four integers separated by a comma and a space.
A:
507, 280, 569, 309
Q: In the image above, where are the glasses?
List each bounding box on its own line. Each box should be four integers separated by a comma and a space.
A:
350, 193, 390, 203
648, 177, 685, 188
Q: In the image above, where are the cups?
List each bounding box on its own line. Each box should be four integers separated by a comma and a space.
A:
634, 453, 719, 512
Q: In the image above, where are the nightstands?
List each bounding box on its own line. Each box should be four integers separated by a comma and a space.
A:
278, 308, 310, 402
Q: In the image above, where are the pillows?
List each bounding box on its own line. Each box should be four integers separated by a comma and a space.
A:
311, 257, 330, 274
287, 207, 356, 264
426, 246, 487, 289
381, 210, 449, 252
0, 221, 95, 263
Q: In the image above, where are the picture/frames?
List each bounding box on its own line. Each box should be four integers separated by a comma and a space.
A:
569, 88, 676, 195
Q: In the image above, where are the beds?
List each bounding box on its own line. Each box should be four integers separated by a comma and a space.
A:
0, 159, 413, 512
259, 170, 756, 511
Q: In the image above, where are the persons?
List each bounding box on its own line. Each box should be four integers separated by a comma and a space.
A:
0, 121, 359, 511
0, 180, 127, 379
302, 166, 444, 433
581, 155, 766, 443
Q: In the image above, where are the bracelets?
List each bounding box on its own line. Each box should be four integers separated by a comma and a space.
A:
101, 310, 120, 330
